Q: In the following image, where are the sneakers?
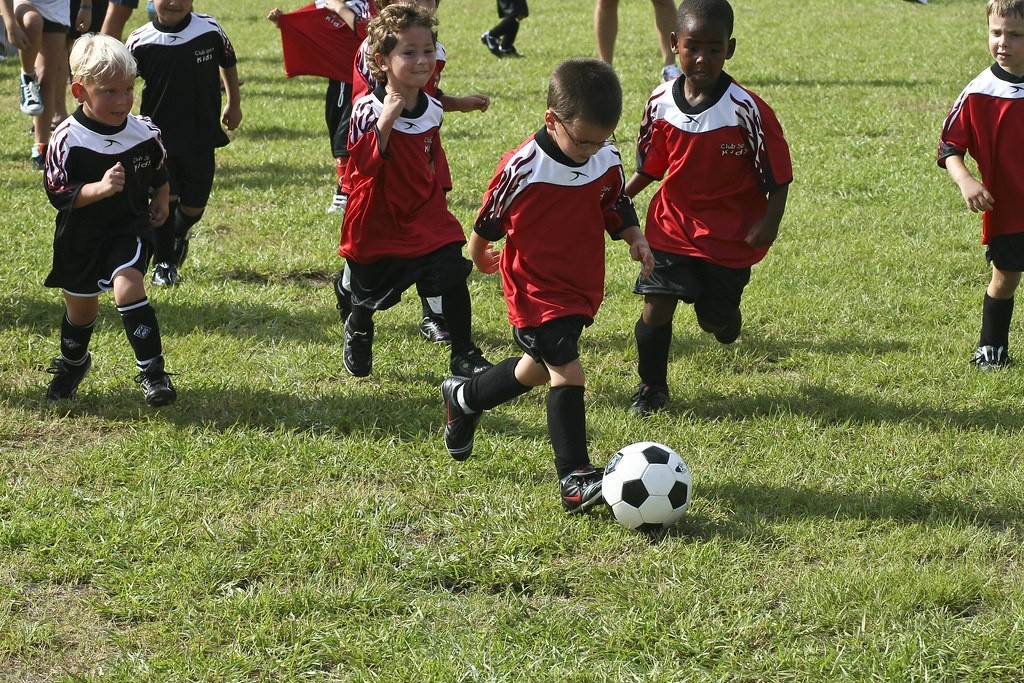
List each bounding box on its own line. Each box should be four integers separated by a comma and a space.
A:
559, 464, 604, 515
481, 31, 502, 57
343, 313, 373, 377
151, 262, 178, 287
134, 357, 177, 407
500, 46, 522, 58
714, 312, 741, 344
418, 316, 452, 344
450, 343, 494, 380
442, 377, 482, 461
18, 75, 44, 115
30, 143, 48, 173
632, 383, 670, 417
327, 194, 347, 215
46, 353, 91, 400
334, 269, 353, 324
970, 345, 1010, 370
661, 64, 682, 85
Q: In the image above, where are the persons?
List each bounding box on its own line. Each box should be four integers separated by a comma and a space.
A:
0, 0, 242, 173
594, 0, 682, 85
264, 1, 389, 214
623, 2, 796, 418
935, 1, 1024, 365
43, 33, 180, 408
333, 0, 495, 378
480, 0, 529, 58
127, 1, 242, 290
442, 62, 650, 515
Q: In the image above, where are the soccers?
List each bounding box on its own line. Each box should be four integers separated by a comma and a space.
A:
602, 440, 694, 535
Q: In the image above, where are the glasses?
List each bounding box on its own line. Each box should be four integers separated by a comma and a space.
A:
554, 115, 617, 150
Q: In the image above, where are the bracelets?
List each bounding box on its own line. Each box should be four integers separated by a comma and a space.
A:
80, 4, 93, 10
